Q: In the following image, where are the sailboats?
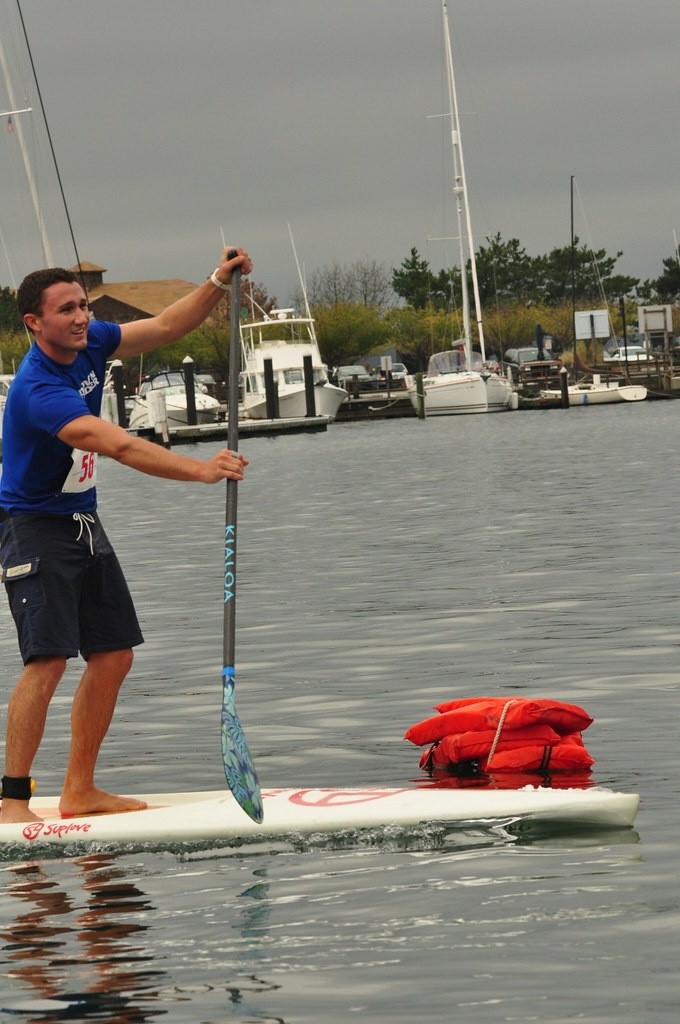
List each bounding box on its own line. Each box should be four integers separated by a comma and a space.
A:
540, 173, 648, 405
0, 50, 120, 426
408, 0, 523, 416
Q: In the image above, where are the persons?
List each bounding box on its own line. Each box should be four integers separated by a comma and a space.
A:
0, 246, 248, 824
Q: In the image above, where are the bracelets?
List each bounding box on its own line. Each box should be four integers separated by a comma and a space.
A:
211, 269, 231, 290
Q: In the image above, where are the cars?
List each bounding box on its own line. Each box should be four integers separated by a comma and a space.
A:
613, 345, 655, 362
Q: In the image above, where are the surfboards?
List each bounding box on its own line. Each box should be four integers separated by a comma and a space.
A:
0, 786, 644, 849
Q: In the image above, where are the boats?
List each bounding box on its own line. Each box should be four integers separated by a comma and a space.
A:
129, 369, 220, 429
0, 373, 16, 463
218, 221, 350, 424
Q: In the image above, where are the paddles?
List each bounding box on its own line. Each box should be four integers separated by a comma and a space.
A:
218, 248, 265, 825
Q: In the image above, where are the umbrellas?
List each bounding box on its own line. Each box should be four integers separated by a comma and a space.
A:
536, 324, 545, 361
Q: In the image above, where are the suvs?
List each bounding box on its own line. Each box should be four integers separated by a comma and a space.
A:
500, 348, 559, 382
329, 365, 374, 391
373, 364, 408, 387
